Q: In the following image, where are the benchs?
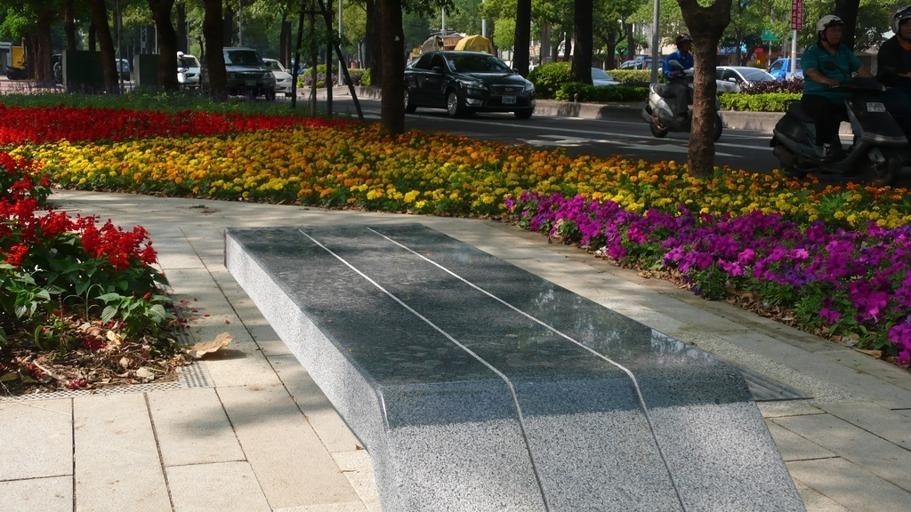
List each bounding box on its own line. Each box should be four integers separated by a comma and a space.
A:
222, 221, 810, 512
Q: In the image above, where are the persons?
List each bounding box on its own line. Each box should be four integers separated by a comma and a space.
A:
175, 50, 188, 73
872, 2, 910, 137
661, 31, 694, 120
800, 11, 876, 165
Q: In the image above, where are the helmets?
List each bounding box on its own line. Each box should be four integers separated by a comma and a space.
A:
891, 5, 911, 34
815, 15, 844, 39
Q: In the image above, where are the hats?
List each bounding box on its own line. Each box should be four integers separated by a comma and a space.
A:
676, 33, 692, 46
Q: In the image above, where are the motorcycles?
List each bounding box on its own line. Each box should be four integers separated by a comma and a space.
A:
640, 59, 723, 142
769, 61, 910, 187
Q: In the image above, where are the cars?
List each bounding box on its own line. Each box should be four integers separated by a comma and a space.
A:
173, 46, 299, 101
714, 65, 776, 97
403, 49, 537, 119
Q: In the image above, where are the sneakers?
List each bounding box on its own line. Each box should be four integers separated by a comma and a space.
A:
816, 144, 832, 162
